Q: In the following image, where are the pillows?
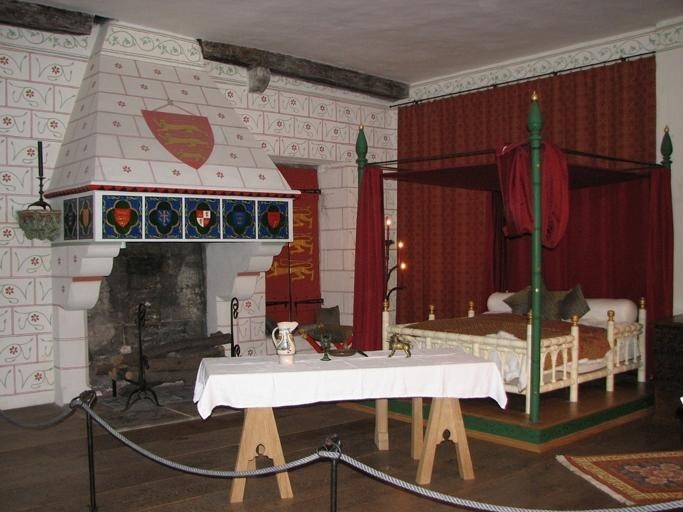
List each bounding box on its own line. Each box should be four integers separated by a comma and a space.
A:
486, 282, 638, 328
313, 305, 339, 326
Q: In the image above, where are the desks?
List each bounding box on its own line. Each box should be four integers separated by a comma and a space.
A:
192, 350, 497, 503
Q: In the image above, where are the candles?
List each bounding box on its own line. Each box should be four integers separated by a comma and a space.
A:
37, 141, 43, 177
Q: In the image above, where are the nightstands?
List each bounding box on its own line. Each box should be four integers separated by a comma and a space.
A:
649, 314, 683, 432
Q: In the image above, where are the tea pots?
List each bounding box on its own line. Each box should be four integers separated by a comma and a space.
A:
271, 321, 299, 364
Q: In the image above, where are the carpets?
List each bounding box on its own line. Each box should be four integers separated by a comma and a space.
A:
552, 448, 682, 512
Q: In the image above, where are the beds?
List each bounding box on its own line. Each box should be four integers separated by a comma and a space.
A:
382, 290, 647, 416
